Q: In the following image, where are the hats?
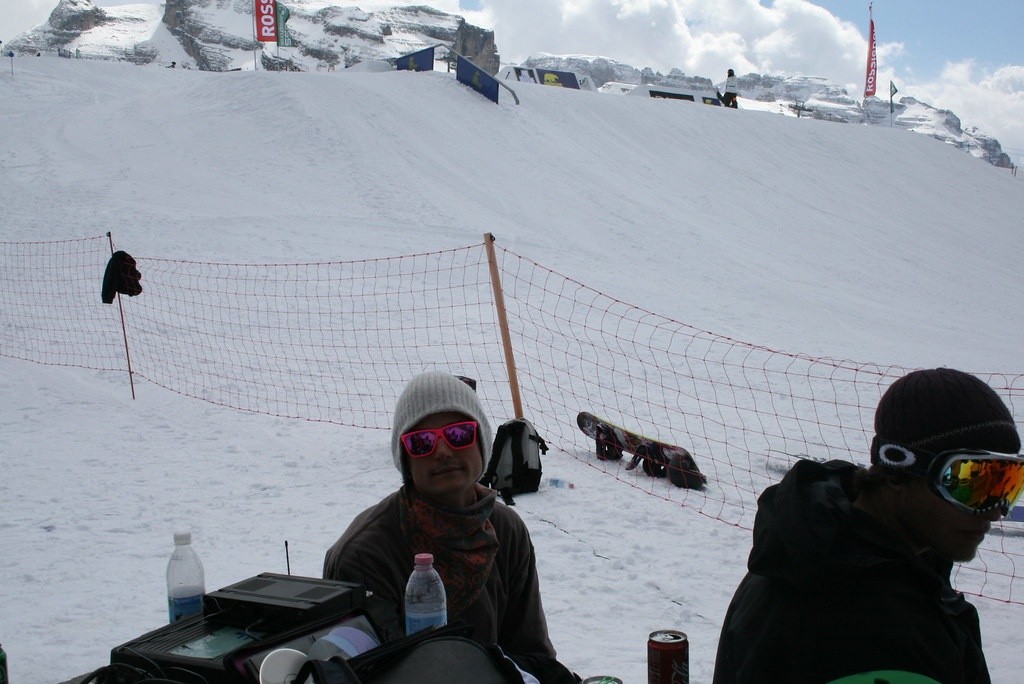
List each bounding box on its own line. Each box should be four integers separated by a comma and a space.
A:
391, 370, 493, 483
875, 367, 1020, 479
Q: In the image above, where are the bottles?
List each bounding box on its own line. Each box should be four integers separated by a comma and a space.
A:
165, 531, 204, 623
405, 553, 447, 635
0, 644, 8, 684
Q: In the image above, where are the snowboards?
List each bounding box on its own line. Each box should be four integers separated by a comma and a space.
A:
576, 411, 701, 474
716, 90, 725, 104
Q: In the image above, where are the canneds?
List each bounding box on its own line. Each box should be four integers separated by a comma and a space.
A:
583, 676, 623, 684
647, 630, 689, 684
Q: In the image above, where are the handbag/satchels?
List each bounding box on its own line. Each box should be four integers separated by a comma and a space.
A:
307, 622, 540, 684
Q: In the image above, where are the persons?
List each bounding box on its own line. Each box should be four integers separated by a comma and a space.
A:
713, 366, 1024, 684
323, 373, 587, 684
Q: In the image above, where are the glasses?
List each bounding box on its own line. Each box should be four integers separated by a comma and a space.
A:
401, 422, 478, 458
925, 449, 1024, 516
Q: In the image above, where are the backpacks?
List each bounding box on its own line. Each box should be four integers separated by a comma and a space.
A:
478, 418, 549, 506
